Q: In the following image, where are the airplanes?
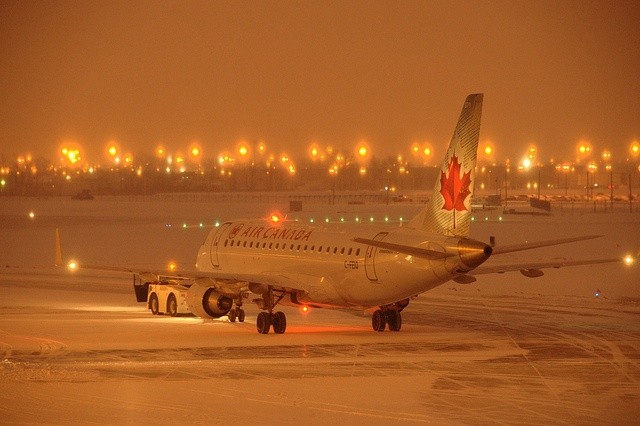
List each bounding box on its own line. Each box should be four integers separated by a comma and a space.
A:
185, 91, 623, 335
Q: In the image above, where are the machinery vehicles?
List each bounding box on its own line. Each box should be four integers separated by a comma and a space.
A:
128, 268, 197, 318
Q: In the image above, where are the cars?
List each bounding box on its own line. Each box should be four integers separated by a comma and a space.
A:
393, 194, 412, 204
505, 193, 639, 205
